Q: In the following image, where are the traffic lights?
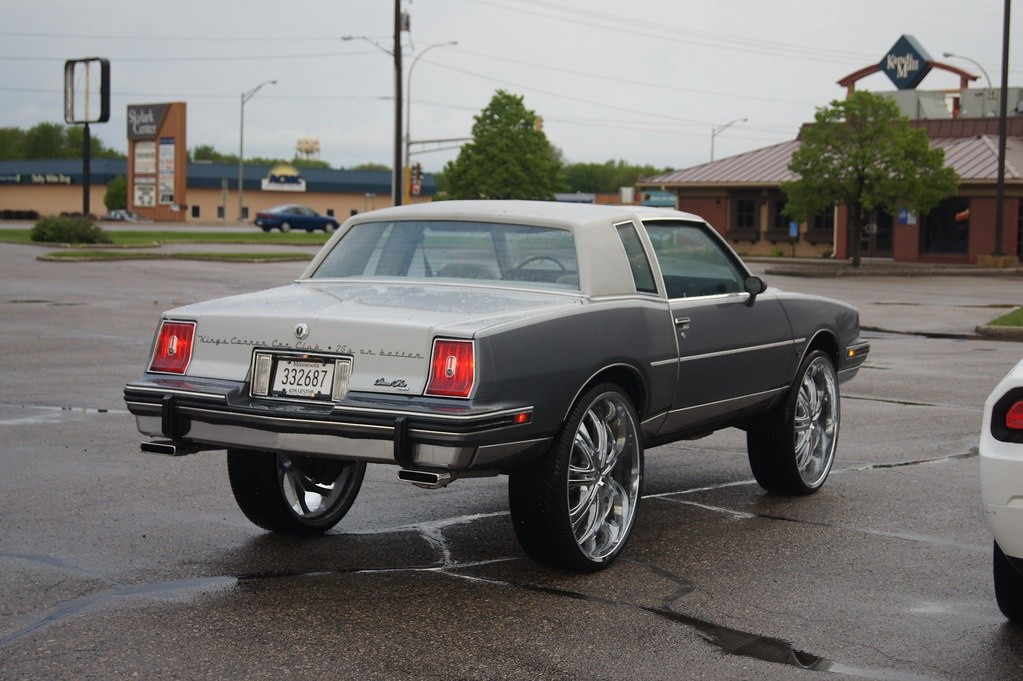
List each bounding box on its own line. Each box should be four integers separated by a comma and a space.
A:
535, 116, 544, 129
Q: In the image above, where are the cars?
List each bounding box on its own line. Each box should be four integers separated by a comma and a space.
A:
253, 204, 340, 233
979, 358, 1023, 618
106, 210, 153, 222
123, 200, 870, 572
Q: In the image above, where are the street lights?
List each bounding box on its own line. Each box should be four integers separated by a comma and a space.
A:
944, 52, 992, 89
343, 35, 401, 205
239, 80, 277, 223
711, 119, 748, 160
404, 42, 458, 204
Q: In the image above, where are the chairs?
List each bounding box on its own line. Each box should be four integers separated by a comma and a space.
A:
556, 274, 579, 286
439, 264, 500, 280
